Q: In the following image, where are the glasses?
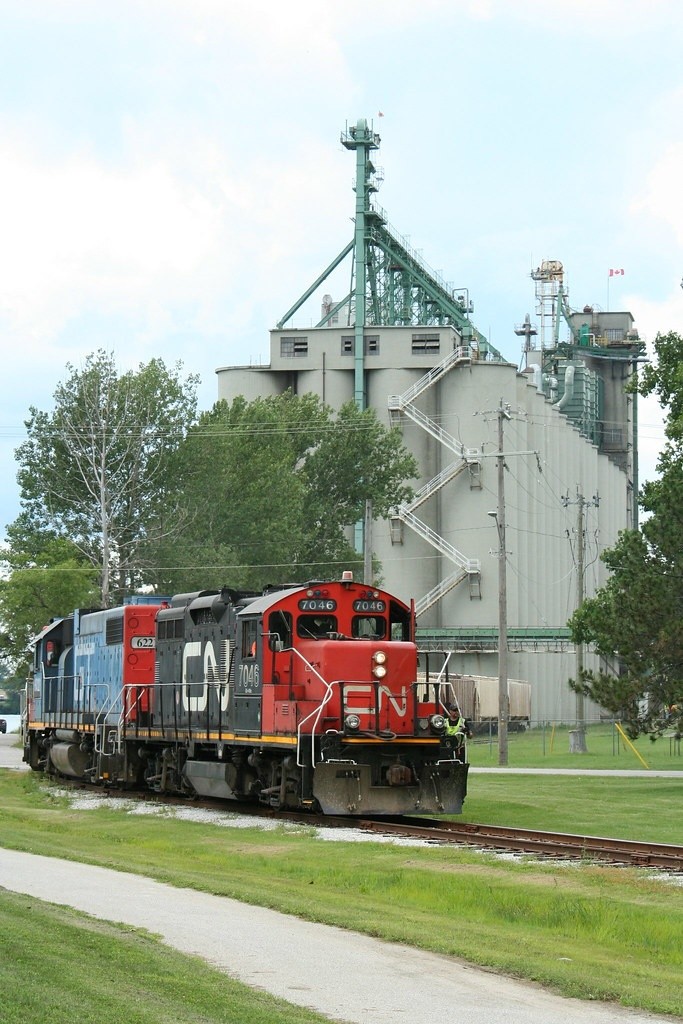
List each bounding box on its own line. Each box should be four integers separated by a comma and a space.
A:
451, 709, 458, 712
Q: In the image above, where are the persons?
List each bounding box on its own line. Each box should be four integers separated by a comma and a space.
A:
444, 703, 474, 806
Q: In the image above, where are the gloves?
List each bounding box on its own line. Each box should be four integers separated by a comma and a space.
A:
463, 727, 470, 735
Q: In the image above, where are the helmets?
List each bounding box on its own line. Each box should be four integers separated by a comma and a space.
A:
449, 704, 458, 709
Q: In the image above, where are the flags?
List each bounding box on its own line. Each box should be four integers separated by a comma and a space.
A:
609, 268, 625, 277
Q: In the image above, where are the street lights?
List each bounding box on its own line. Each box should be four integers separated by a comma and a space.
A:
486, 511, 508, 765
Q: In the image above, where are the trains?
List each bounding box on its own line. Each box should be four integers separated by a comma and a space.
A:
20, 569, 531, 820
